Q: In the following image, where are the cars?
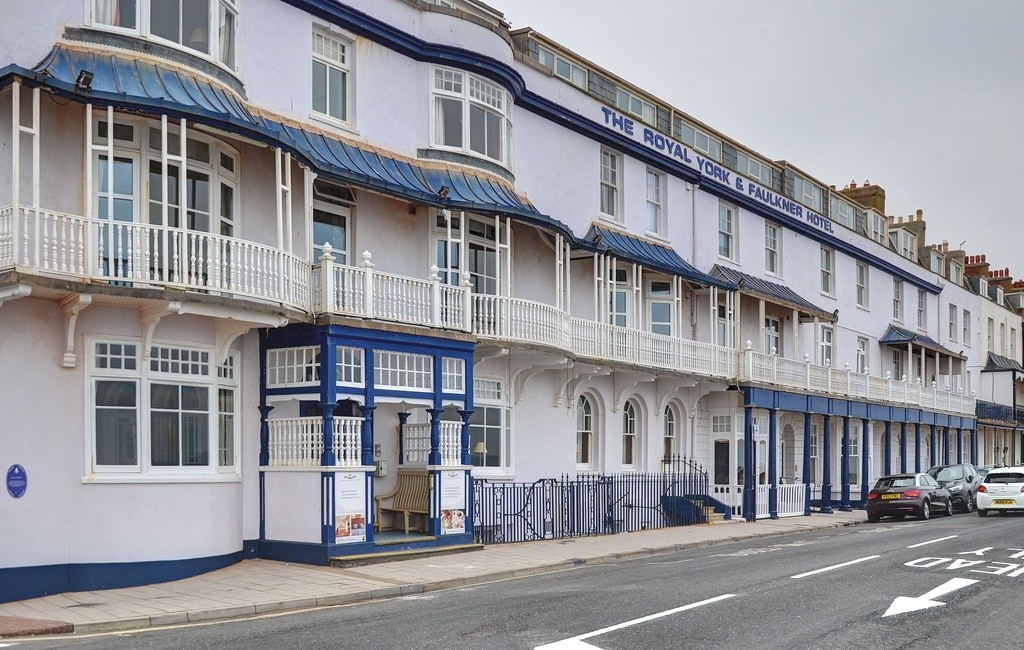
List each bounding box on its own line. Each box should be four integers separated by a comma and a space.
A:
975, 466, 1024, 517
928, 462, 978, 513
866, 472, 954, 519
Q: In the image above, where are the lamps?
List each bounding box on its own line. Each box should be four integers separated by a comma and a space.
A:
739, 277, 745, 289
435, 184, 450, 203
74, 69, 96, 95
473, 442, 487, 465
591, 234, 603, 247
832, 308, 839, 317
911, 334, 918, 341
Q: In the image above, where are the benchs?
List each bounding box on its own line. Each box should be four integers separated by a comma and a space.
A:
375, 470, 431, 535
